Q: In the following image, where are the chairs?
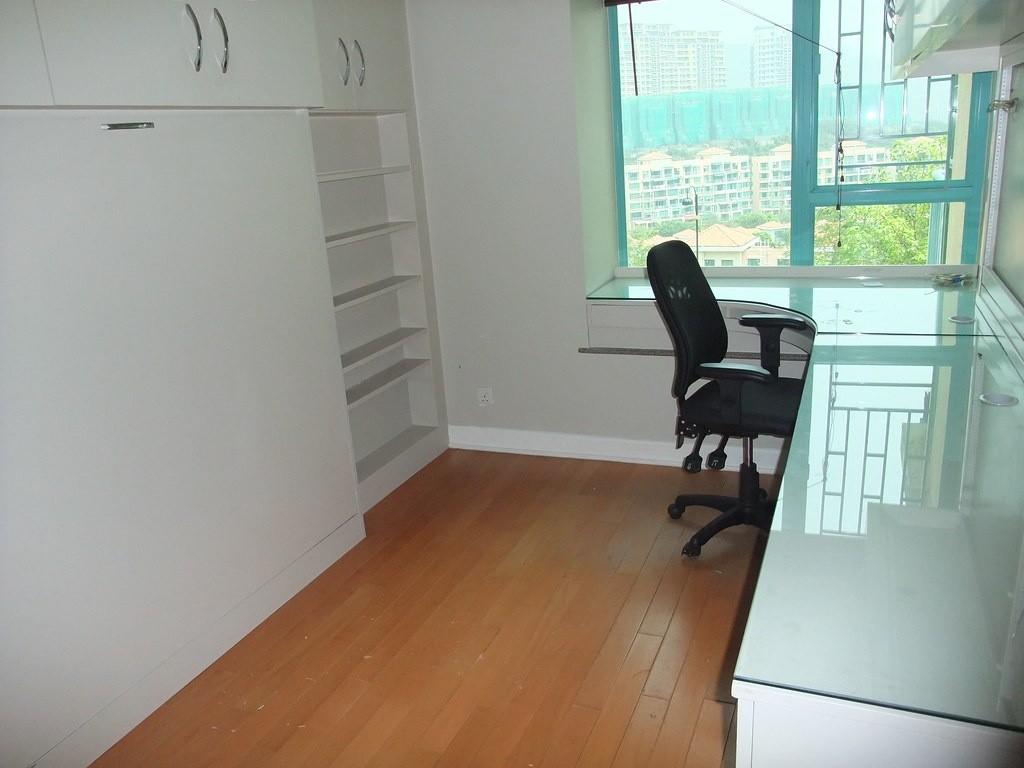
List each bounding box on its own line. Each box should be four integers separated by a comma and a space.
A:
646, 239, 808, 558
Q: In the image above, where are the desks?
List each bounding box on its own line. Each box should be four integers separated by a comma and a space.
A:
586, 276, 1024, 767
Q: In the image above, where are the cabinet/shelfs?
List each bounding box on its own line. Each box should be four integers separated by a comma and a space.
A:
0, 0, 54, 110
308, 108, 450, 514
309, 0, 415, 116
0, 108, 368, 767
34, 0, 325, 109
